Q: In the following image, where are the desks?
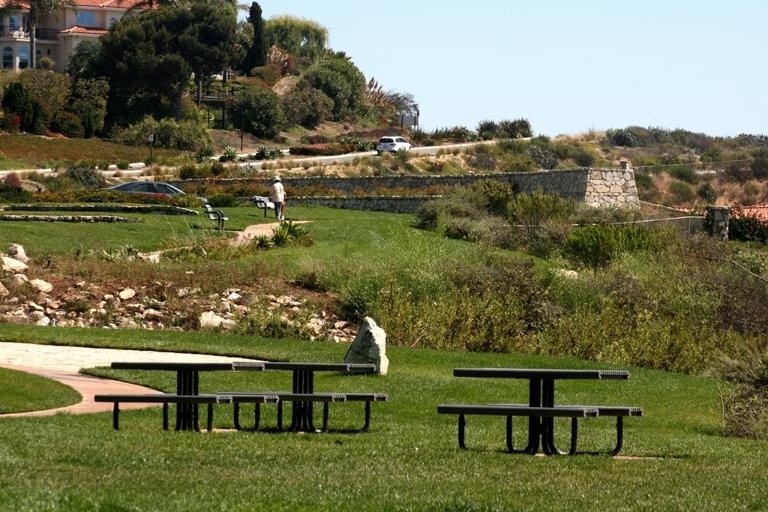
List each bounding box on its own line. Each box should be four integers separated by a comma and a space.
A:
111, 358, 265, 431
265, 362, 378, 431
454, 368, 628, 454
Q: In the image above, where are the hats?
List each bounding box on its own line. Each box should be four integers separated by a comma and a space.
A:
272, 176, 281, 182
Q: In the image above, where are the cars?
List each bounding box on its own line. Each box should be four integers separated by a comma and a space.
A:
96, 181, 208, 208
376, 136, 411, 155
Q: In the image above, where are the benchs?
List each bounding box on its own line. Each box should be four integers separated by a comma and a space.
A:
251, 196, 275, 218
439, 401, 645, 458
95, 392, 388, 433
205, 204, 228, 231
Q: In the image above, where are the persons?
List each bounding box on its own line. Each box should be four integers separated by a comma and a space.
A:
269, 176, 285, 219
280, 191, 287, 221
